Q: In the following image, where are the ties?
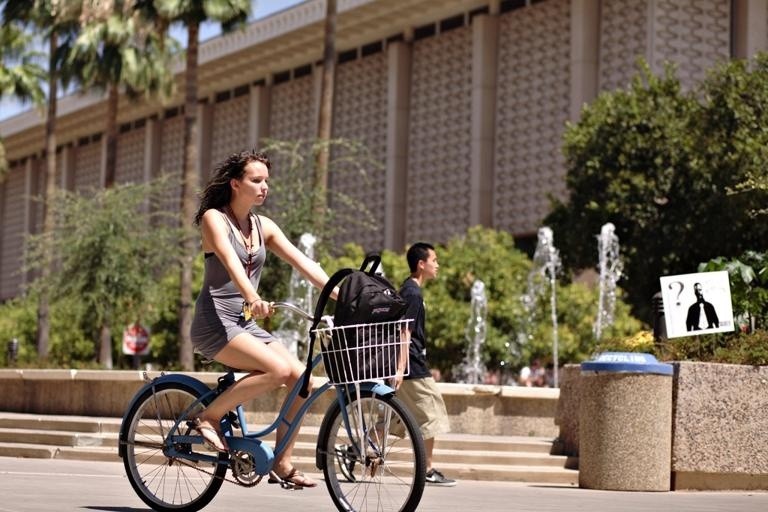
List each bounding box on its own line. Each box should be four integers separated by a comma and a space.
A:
698, 303, 709, 330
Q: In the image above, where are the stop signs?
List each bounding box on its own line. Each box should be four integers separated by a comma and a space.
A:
122, 323, 151, 355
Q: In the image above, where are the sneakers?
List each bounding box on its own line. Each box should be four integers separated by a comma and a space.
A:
425, 468, 456, 486
334, 443, 356, 482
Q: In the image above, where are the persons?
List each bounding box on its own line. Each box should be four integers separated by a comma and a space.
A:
686, 283, 719, 331
334, 243, 459, 487
186, 148, 340, 487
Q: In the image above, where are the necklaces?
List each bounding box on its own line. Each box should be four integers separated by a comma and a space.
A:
227, 203, 252, 280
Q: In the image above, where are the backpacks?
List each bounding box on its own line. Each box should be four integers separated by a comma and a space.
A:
298, 254, 409, 398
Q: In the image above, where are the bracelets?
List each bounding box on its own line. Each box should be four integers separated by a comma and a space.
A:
250, 298, 262, 307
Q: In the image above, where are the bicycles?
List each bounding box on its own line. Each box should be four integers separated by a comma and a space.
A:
119, 301, 426, 512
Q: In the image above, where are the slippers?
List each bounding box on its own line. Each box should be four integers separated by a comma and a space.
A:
186, 420, 231, 454
268, 467, 317, 487
